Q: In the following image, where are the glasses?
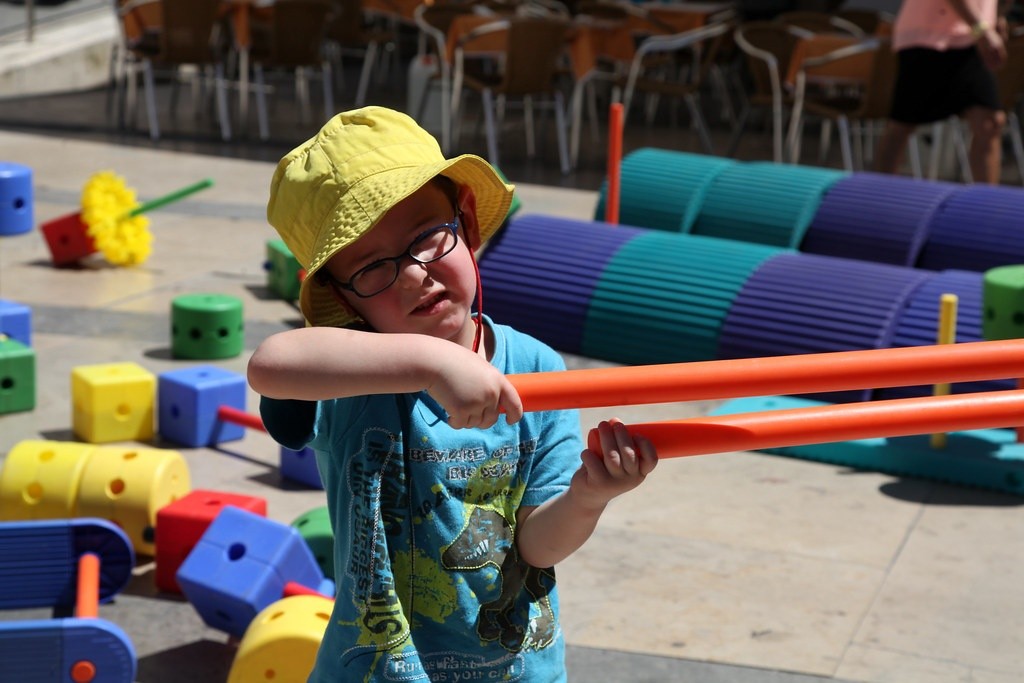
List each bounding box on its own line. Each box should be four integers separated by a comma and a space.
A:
330, 204, 460, 298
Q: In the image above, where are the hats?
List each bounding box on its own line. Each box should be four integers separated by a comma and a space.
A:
268, 107, 516, 324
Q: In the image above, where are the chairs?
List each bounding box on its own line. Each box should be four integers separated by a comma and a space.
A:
105, 0, 1024, 191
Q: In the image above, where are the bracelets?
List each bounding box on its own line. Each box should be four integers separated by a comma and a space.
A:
970, 20, 991, 40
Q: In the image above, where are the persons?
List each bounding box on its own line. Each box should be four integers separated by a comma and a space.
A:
872, 0, 1007, 184
247, 106, 657, 683
